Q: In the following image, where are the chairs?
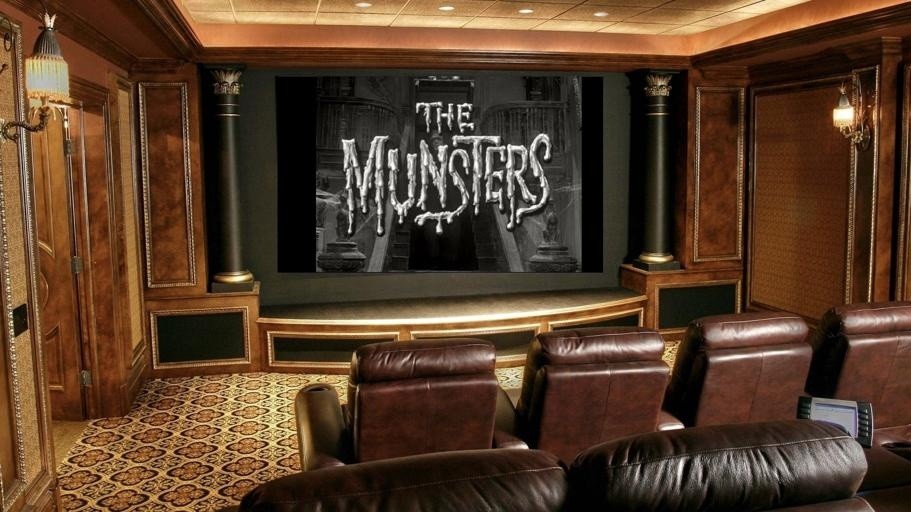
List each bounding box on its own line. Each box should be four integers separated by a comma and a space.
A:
514, 325, 671, 454
291, 336, 529, 474
573, 419, 876, 511
808, 299, 911, 429
662, 311, 809, 421
222, 444, 570, 512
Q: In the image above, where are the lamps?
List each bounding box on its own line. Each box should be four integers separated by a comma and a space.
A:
2, 6, 72, 143
833, 82, 865, 146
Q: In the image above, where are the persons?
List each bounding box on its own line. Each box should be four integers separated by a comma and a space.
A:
408, 129, 481, 271
